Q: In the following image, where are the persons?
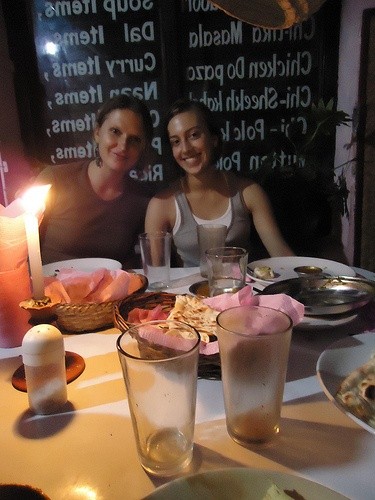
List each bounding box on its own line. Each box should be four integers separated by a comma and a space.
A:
145, 101, 296, 268
0, 95, 154, 269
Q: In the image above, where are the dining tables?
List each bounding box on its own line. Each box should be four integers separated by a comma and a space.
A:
0, 266, 375, 500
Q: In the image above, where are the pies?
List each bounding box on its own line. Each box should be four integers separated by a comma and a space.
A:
154, 294, 230, 342
334, 356, 375, 422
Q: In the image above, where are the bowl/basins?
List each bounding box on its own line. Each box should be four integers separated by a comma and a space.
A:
262, 275, 375, 315
188, 278, 245, 298
294, 266, 323, 276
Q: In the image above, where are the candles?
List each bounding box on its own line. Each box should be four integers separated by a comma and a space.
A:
20, 180, 45, 302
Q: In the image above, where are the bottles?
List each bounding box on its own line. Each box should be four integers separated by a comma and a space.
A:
21, 324, 67, 415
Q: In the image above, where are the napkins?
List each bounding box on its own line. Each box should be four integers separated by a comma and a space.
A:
126, 284, 304, 357
40, 264, 146, 305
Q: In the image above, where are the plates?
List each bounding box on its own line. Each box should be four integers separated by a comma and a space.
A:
42, 258, 123, 277
246, 257, 356, 286
316, 332, 375, 435
141, 468, 353, 500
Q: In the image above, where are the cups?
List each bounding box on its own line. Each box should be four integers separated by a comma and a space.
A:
204, 247, 248, 298
216, 305, 293, 445
139, 232, 172, 291
197, 225, 227, 278
0, 263, 34, 348
115, 320, 201, 477
